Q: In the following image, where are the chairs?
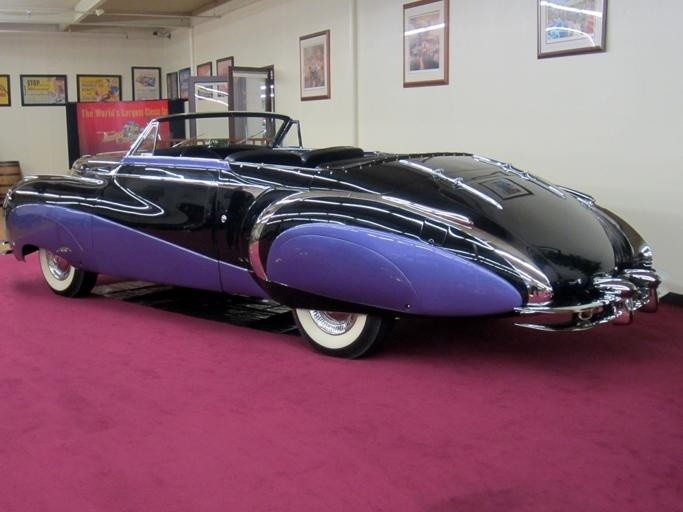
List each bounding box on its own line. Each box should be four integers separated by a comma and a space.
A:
225, 145, 364, 169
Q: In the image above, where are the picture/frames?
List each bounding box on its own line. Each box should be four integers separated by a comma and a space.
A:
297, 29, 333, 100
165, 56, 234, 102
401, 0, 449, 87
131, 66, 161, 100
0, 73, 122, 106
535, 0, 607, 58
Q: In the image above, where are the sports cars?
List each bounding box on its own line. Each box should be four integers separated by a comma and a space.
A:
0, 110, 663, 360
